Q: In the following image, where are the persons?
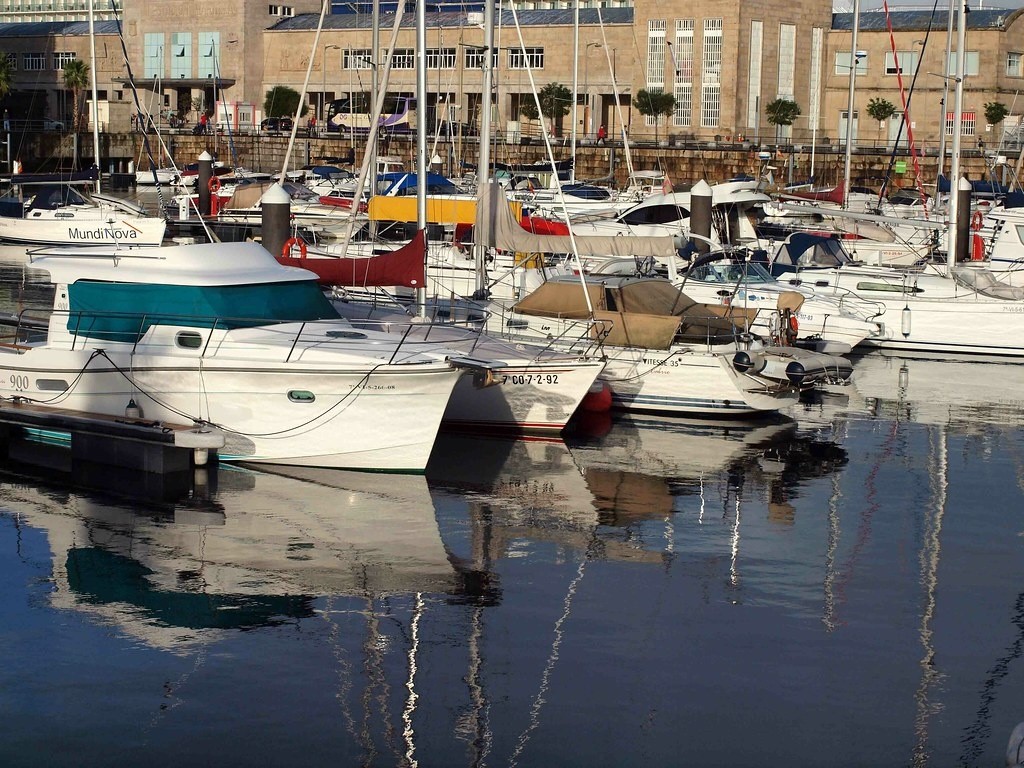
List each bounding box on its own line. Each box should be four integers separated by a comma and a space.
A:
597, 125, 606, 144
978, 137, 984, 156
308, 116, 316, 131
199, 112, 206, 135
170, 115, 184, 128
131, 114, 144, 132
621, 125, 627, 146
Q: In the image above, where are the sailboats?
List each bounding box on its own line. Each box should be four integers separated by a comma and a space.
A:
1, 1, 1024, 436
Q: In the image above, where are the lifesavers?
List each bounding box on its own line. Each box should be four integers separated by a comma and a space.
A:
280, 237, 308, 260
774, 311, 800, 346
971, 210, 984, 232
207, 176, 221, 192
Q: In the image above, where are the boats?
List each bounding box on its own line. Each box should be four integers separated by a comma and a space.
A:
0, 415, 489, 768
0, 242, 467, 469
